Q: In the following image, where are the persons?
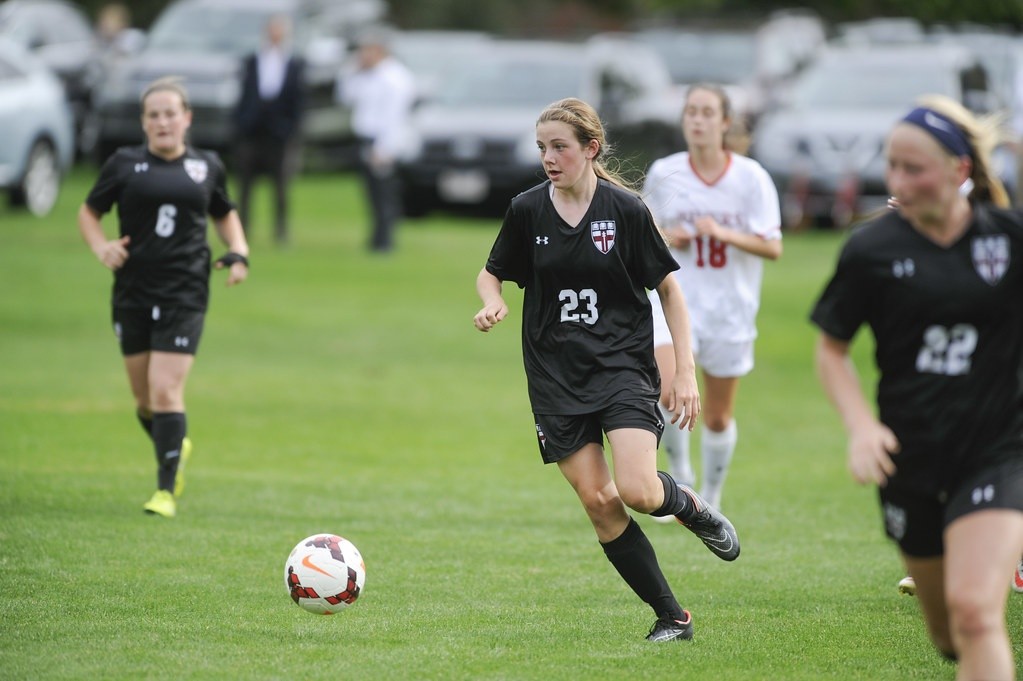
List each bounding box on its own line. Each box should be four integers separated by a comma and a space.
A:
344, 29, 421, 251
77, 74, 252, 516
637, 82, 784, 531
808, 94, 1023, 681
473, 95, 742, 644
885, 165, 1023, 598
223, 13, 310, 246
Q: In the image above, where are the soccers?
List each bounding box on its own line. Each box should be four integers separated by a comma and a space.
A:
285, 534, 366, 616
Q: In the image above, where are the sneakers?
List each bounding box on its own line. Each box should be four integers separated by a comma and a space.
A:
677, 483, 740, 562
144, 488, 176, 519
1013, 561, 1023, 593
174, 437, 191, 496
645, 610, 693, 643
899, 576, 918, 596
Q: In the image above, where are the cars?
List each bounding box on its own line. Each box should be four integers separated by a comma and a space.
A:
0, 0, 1022, 238
